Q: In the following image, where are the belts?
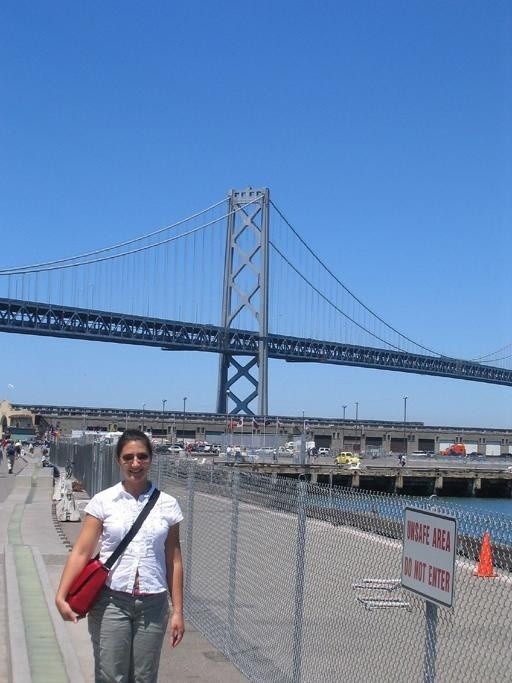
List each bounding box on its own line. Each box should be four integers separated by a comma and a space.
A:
104, 584, 154, 596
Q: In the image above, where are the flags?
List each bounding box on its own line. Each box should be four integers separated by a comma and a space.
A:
228, 415, 312, 431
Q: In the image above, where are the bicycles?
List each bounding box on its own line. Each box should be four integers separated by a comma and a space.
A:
65, 459, 74, 478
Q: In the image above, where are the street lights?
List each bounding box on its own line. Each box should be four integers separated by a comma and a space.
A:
143, 404, 146, 431
161, 400, 167, 444
402, 397, 408, 450
342, 406, 347, 444
183, 397, 187, 449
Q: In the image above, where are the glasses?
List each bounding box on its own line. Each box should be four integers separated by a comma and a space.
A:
119, 452, 151, 463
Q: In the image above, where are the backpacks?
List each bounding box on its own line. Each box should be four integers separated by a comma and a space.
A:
7, 445, 14, 455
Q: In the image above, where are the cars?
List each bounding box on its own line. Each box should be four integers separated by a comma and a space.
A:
255, 447, 274, 455
411, 450, 435, 458
334, 452, 360, 464
156, 443, 221, 453
318, 447, 330, 455
471, 450, 487, 460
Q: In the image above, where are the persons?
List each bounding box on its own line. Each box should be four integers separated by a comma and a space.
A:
54, 429, 186, 682
1, 433, 50, 475
226, 441, 406, 466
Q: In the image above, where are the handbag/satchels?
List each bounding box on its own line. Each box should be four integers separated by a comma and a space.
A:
65, 558, 109, 617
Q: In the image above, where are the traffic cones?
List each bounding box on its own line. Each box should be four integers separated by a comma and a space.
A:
473, 528, 498, 577
